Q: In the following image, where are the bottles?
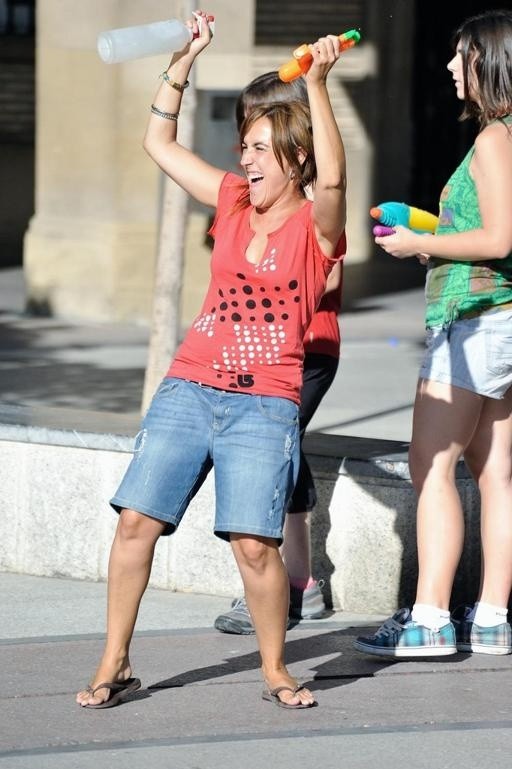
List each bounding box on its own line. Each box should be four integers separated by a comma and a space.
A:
98, 19, 216, 62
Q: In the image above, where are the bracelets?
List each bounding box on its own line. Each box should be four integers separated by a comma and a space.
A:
162, 71, 189, 92
151, 103, 178, 120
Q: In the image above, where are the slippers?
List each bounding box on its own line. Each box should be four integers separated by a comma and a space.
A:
261, 682, 318, 710
80, 678, 141, 709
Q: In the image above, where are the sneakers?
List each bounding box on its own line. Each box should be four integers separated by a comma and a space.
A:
289, 580, 326, 622
352, 607, 459, 657
446, 610, 512, 655
214, 592, 256, 634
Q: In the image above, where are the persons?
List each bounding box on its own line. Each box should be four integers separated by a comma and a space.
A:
76, 8, 347, 709
353, 10, 512, 657
214, 72, 343, 636
